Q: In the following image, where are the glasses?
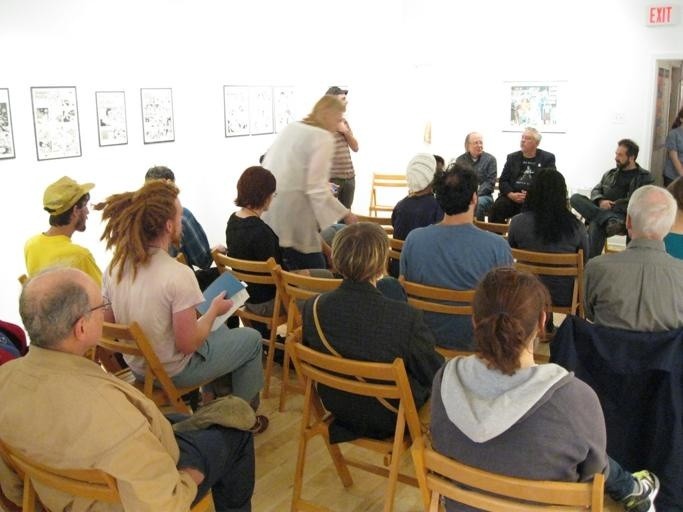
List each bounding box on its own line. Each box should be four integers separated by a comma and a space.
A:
73, 295, 112, 328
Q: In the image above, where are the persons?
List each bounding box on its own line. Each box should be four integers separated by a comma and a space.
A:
24, 176, 104, 301
301, 221, 449, 444
260, 86, 359, 271
0, 320, 29, 367
147, 166, 239, 330
227, 165, 296, 371
662, 106, 683, 209
429, 266, 660, 512
94, 179, 269, 437
388, 127, 682, 346
1, 268, 256, 511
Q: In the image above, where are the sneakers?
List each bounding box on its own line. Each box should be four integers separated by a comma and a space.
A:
622, 470, 660, 512
248, 415, 268, 434
606, 220, 624, 234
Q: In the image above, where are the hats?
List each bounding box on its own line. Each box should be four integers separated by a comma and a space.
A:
173, 396, 257, 434
326, 87, 348, 94
43, 176, 94, 217
406, 153, 436, 193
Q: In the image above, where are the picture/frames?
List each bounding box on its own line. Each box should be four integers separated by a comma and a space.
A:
222, 84, 302, 138
0, 84, 176, 164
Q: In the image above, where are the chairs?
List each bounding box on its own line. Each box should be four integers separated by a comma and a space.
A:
209, 246, 301, 400
405, 432, 605, 512
1, 440, 217, 511
79, 311, 220, 425
267, 263, 347, 414
2, 167, 636, 319
393, 275, 485, 362
283, 339, 423, 510
506, 246, 589, 373
